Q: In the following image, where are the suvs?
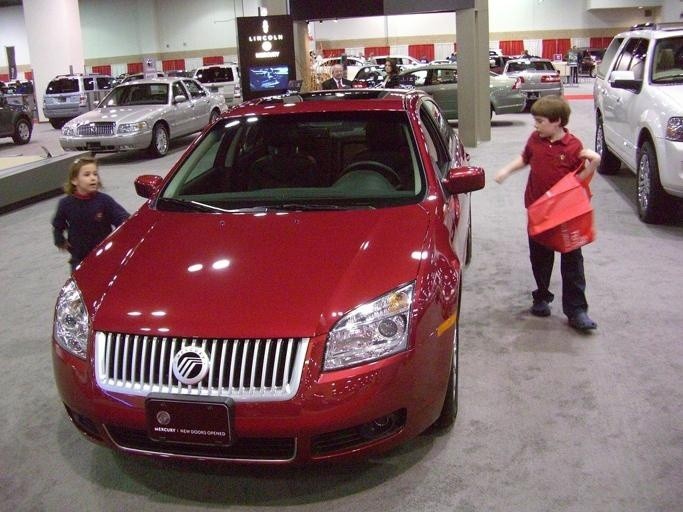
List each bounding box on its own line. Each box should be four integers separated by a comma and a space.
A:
591, 20, 683, 229
42, 72, 122, 130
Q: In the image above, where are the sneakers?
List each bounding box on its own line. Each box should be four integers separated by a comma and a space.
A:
568, 312, 597, 330
528, 300, 551, 316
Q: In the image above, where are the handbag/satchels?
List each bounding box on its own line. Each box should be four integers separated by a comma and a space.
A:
527, 159, 596, 254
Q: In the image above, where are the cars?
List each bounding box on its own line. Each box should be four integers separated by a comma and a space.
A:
49, 87, 487, 476
59, 76, 230, 158
312, 47, 459, 88
386, 64, 528, 122
116, 69, 195, 86
573, 46, 606, 78
502, 58, 564, 102
0, 90, 36, 146
193, 65, 242, 111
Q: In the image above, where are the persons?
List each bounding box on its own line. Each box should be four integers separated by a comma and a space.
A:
14, 80, 25, 93
49, 156, 131, 277
320, 64, 354, 92
379, 56, 402, 88
493, 94, 602, 331
